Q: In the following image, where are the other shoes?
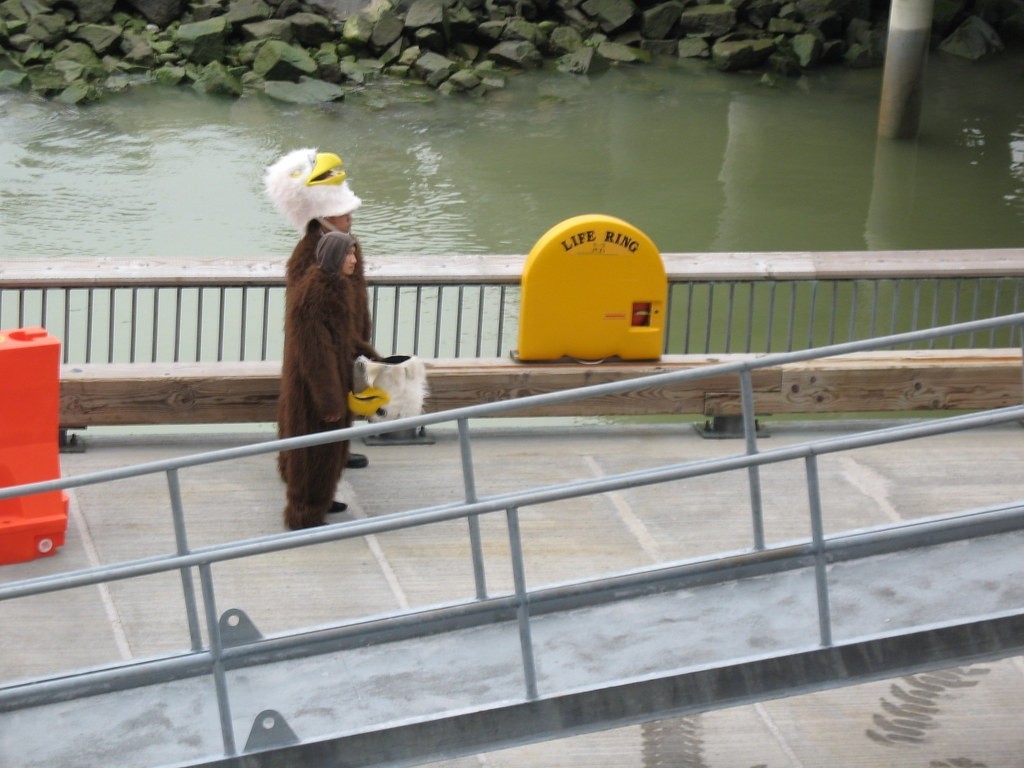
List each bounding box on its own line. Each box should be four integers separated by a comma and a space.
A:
329, 501, 348, 513
344, 453, 369, 469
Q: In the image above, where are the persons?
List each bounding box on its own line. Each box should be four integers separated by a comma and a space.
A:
263, 147, 378, 481
284, 232, 357, 532
